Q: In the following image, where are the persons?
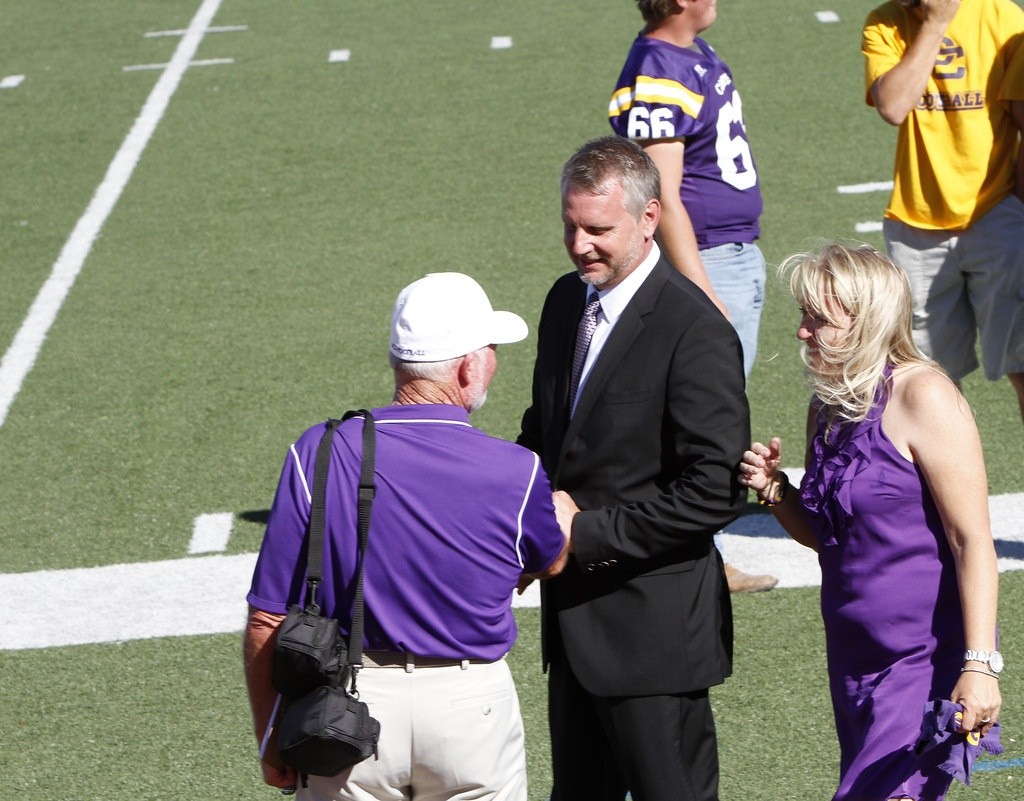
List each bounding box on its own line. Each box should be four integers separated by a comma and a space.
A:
244, 273, 568, 801
860, 0, 1024, 426
516, 136, 751, 801
608, 0, 766, 379
737, 239, 1004, 801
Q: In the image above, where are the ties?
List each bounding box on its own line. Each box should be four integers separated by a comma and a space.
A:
568, 293, 602, 423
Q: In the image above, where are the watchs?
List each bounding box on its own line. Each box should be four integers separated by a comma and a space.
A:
963, 650, 1004, 673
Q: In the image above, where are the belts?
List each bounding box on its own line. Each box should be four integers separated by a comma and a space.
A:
361, 651, 486, 668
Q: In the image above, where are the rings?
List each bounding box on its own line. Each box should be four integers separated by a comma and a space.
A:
982, 717, 990, 722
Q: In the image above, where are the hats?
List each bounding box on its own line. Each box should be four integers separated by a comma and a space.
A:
389, 272, 529, 362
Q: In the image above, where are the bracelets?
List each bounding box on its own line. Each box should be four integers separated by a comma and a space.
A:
757, 471, 789, 507
961, 667, 999, 680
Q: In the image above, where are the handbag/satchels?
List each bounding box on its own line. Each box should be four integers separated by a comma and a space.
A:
271, 408, 380, 788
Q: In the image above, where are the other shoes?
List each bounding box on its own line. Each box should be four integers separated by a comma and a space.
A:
724, 562, 779, 595
